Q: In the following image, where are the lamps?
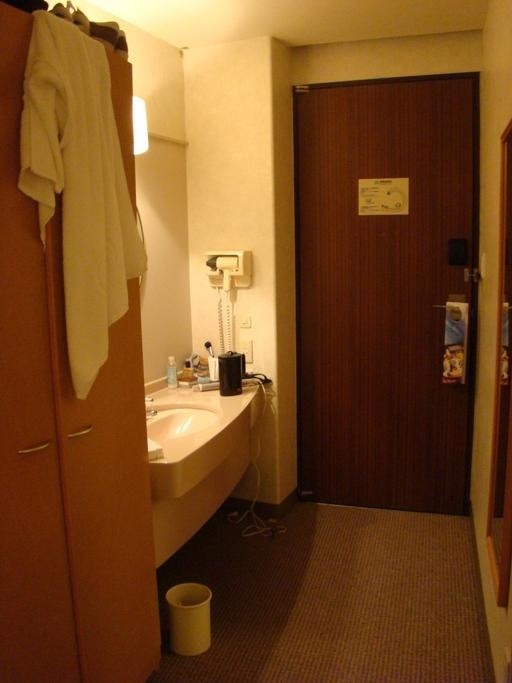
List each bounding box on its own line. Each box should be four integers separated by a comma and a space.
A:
133, 96, 150, 157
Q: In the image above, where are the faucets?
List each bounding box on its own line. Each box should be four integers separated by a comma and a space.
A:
145, 397, 157, 417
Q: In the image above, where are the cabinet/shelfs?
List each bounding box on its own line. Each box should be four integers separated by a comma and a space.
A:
0, 2, 164, 683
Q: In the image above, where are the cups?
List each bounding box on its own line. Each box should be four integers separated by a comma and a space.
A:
218, 350, 247, 396
208, 356, 219, 381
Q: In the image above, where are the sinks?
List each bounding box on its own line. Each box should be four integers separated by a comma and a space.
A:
147, 406, 219, 441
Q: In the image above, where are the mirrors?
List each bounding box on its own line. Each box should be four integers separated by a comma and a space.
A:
133, 131, 193, 397
